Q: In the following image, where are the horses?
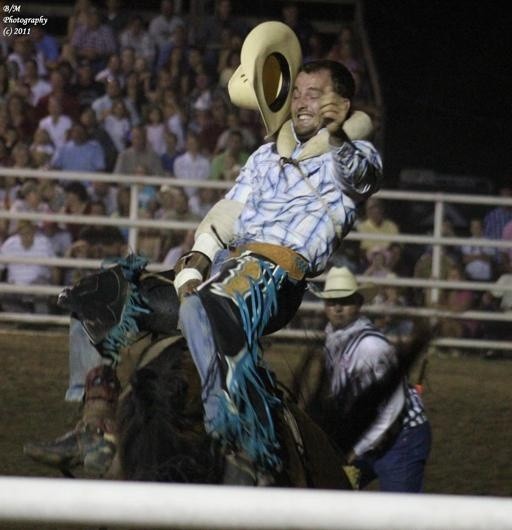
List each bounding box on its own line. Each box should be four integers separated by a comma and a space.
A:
105, 319, 447, 492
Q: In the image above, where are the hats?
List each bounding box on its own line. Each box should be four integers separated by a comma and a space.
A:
306, 265, 375, 299
228, 19, 303, 138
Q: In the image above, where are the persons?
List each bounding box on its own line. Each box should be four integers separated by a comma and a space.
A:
1, 0, 512, 361
15, 59, 388, 486
300, 267, 435, 493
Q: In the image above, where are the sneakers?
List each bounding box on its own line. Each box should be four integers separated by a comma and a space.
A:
23, 434, 113, 474
